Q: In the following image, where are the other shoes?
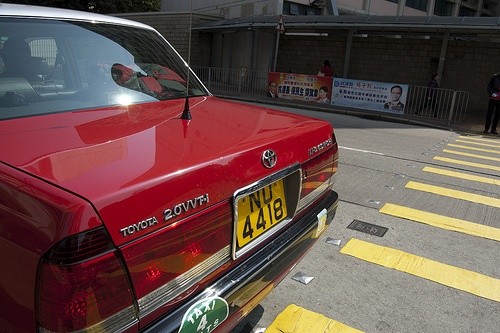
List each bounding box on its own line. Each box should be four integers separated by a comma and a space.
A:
483, 129, 489, 133
491, 128, 498, 135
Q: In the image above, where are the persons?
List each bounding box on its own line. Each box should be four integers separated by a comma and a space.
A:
384, 86, 404, 111
267, 81, 278, 98
1, 37, 31, 76
415, 73, 441, 120
321, 60, 333, 77
317, 86, 330, 104
483, 70, 500, 134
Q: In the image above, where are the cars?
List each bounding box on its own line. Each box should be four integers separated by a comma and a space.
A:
0, 3, 341, 333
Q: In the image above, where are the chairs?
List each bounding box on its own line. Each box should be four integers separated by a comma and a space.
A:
20, 57, 48, 83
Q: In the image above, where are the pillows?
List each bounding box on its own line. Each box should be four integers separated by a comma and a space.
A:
97, 63, 189, 99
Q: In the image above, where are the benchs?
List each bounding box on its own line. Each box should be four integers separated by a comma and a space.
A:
0, 77, 108, 106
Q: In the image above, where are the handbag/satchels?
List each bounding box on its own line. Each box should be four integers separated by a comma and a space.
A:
318, 71, 325, 76
